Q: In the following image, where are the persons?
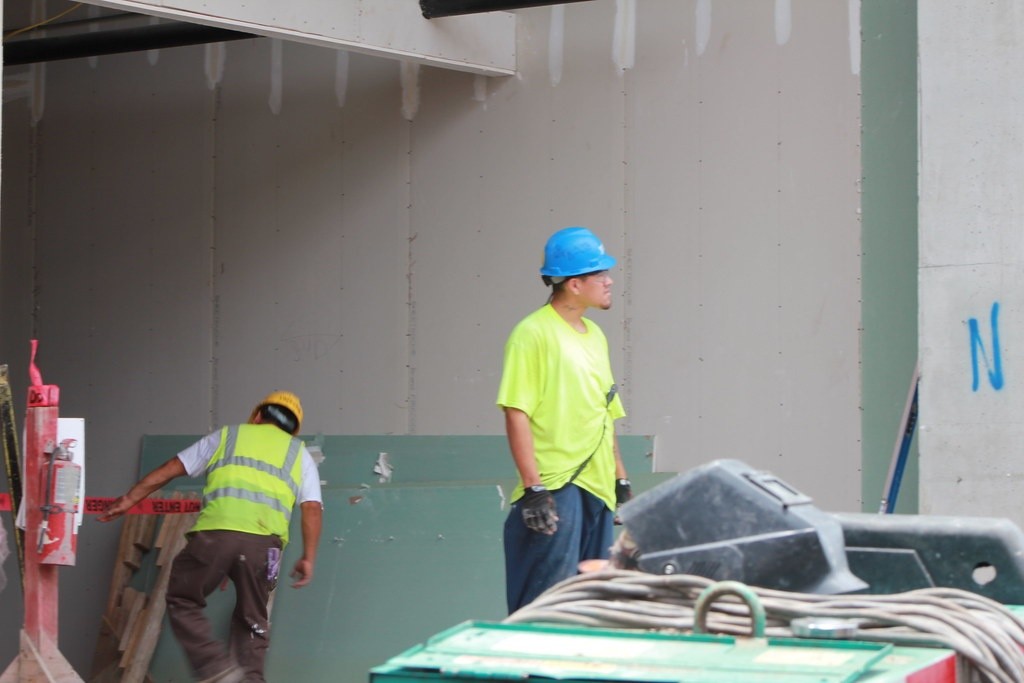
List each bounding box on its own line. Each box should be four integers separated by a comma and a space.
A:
496, 225, 631, 620
95, 391, 324, 683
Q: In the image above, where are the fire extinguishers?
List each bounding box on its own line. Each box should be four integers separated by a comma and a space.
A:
35, 438, 82, 566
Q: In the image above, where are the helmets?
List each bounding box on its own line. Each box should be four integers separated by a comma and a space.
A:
540, 226, 617, 277
247, 391, 303, 436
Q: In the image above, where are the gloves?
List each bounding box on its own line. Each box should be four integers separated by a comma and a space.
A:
522, 485, 559, 536
613, 478, 632, 525
